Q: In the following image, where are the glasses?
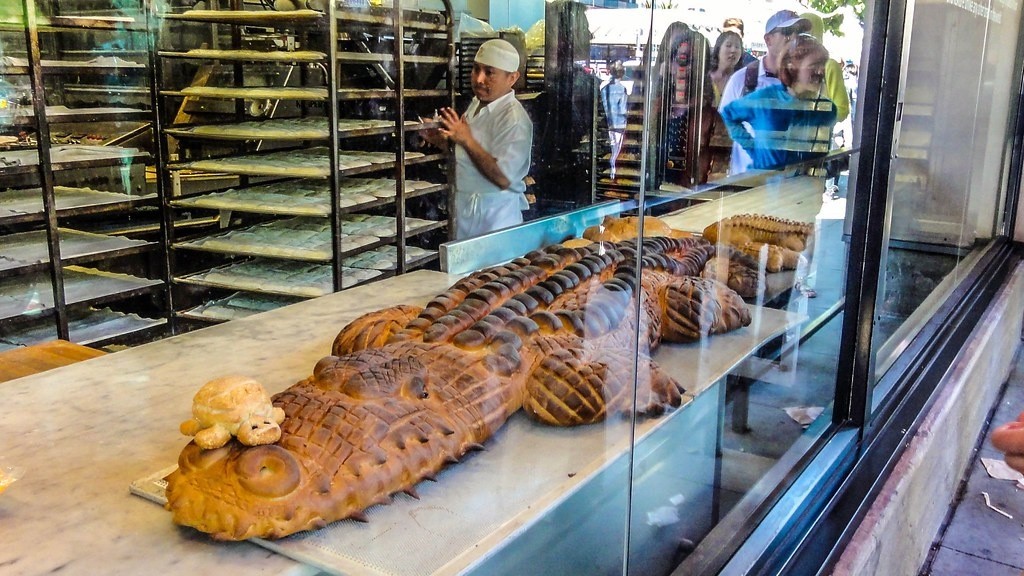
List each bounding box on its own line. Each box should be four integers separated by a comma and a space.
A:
770, 26, 805, 36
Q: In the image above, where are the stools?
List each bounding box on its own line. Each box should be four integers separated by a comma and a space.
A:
0, 339, 110, 386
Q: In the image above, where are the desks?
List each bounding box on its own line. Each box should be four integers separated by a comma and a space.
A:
0, 170, 849, 576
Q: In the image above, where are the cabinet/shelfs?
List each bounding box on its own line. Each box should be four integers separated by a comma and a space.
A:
0, 0, 545, 347
591, 74, 661, 203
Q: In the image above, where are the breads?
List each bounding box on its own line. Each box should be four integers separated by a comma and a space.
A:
180, 9, 647, 323
160, 213, 815, 540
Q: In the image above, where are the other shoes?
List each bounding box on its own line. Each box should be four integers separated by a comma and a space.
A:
798, 283, 817, 298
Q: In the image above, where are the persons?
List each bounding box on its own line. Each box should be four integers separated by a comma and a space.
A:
438, 39, 534, 240
708, 31, 743, 97
823, 59, 858, 200
529, 1, 602, 217
717, 10, 812, 178
799, 13, 850, 150
723, 19, 758, 67
600, 56, 628, 179
721, 33, 837, 298
615, 30, 732, 220
653, 21, 717, 106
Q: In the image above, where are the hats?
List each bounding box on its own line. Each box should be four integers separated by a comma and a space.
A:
765, 9, 812, 35
474, 39, 519, 72
